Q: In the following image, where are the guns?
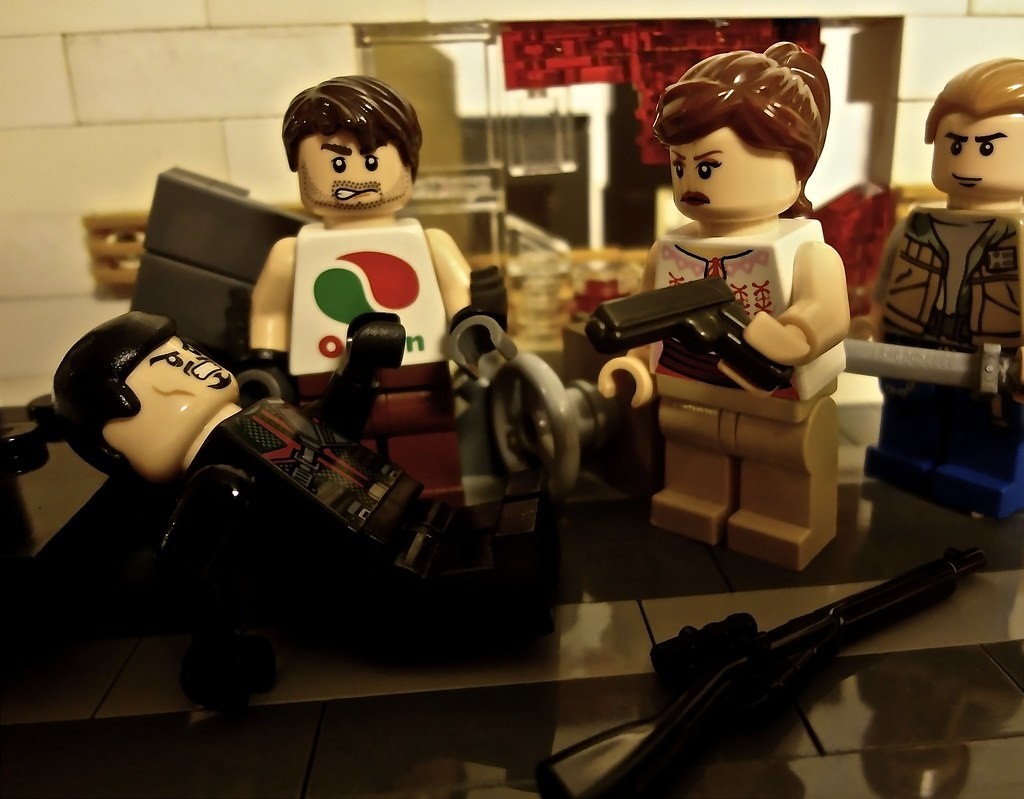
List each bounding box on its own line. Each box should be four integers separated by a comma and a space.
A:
534, 544, 993, 799
583, 275, 795, 393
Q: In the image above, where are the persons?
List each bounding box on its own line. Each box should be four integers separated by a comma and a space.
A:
248, 76, 512, 514
861, 55, 1024, 520
594, 38, 852, 571
51, 309, 566, 716
